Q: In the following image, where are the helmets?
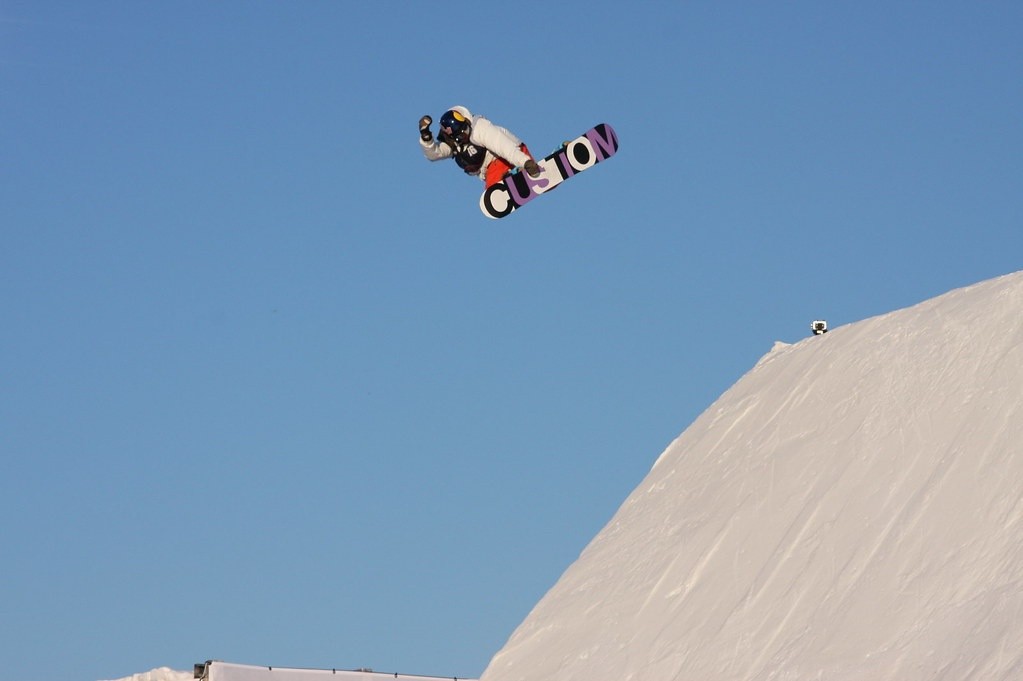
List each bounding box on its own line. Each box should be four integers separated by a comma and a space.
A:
439, 110, 470, 138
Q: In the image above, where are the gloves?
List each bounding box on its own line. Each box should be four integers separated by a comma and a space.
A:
524, 160, 540, 178
418, 115, 433, 141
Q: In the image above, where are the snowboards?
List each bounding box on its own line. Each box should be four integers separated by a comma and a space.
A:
479, 123, 620, 220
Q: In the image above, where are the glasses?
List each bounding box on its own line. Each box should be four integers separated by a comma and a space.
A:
454, 125, 471, 143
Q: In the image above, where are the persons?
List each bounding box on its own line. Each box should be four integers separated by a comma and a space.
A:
419, 106, 541, 190
